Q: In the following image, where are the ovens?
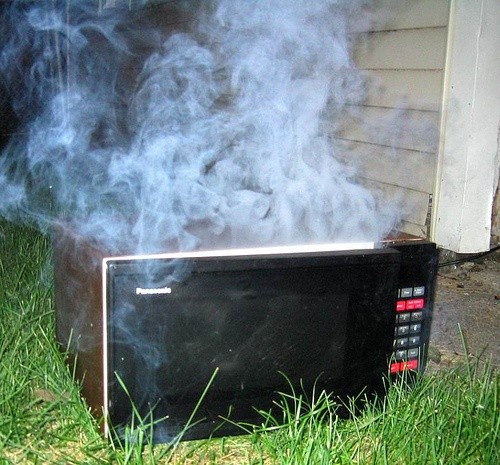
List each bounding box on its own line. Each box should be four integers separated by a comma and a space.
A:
50, 217, 439, 447
